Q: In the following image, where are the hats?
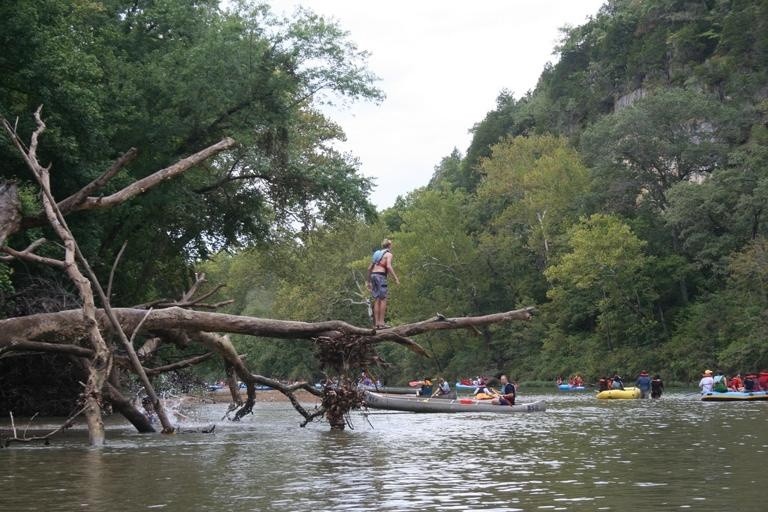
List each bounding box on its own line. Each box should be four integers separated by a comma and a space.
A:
705, 370, 713, 374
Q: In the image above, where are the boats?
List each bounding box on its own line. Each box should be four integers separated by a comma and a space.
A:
558, 383, 586, 391
698, 387, 767, 403
202, 376, 426, 394
594, 386, 640, 401
359, 387, 549, 414
454, 381, 485, 394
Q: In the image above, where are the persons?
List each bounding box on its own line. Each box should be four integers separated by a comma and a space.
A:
557, 377, 562, 384
635, 370, 651, 399
698, 368, 768, 391
652, 374, 664, 398
366, 239, 400, 329
140, 396, 188, 424
439, 377, 451, 395
216, 377, 247, 389
419, 377, 432, 395
460, 374, 519, 404
598, 375, 624, 391
569, 375, 584, 386
359, 372, 384, 387
